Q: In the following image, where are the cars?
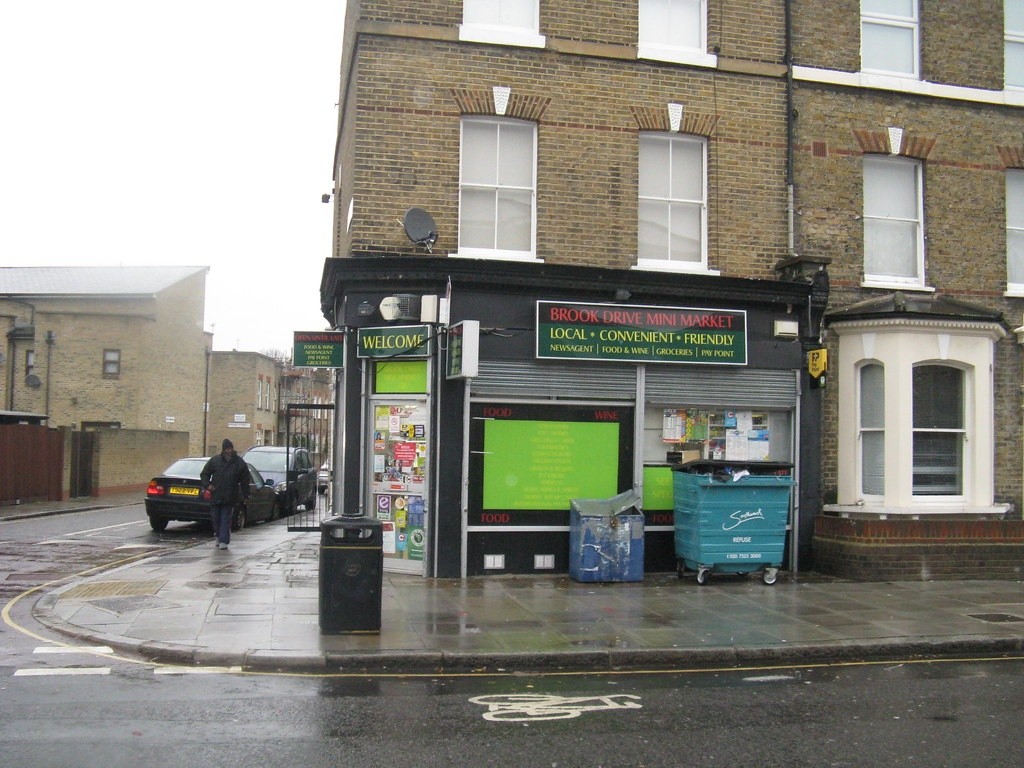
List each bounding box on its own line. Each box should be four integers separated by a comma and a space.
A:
240, 444, 318, 515
144, 456, 280, 532
316, 458, 330, 495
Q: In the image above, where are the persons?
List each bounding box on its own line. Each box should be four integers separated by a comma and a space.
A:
200, 439, 250, 549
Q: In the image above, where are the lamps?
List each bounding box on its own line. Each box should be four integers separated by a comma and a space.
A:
615, 289, 632, 301
358, 302, 375, 316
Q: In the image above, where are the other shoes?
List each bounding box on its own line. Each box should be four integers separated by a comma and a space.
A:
215, 537, 219, 545
218, 543, 228, 549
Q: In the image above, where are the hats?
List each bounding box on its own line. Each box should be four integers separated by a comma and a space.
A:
223, 439, 233, 449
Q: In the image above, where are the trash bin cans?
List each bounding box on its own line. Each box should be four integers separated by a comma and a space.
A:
318, 517, 385, 636
670, 458, 799, 585
569, 491, 646, 585
